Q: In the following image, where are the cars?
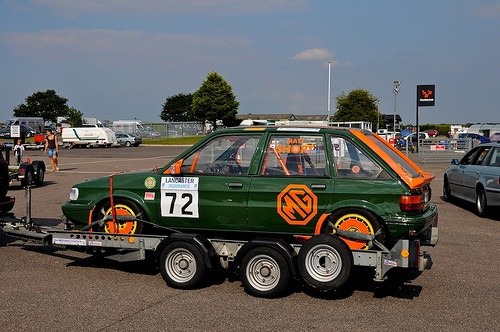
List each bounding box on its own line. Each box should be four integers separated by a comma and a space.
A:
63, 124, 438, 254
0, 123, 37, 139
445, 129, 500, 151
115, 132, 143, 148
443, 142, 500, 218
238, 117, 439, 159
43, 116, 224, 151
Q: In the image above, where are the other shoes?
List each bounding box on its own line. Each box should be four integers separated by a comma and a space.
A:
52, 169, 57, 172
56, 167, 60, 171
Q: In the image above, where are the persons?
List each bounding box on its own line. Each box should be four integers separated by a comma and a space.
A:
44, 129, 60, 171
10, 139, 25, 165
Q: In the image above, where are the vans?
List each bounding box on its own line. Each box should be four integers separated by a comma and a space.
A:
3, 117, 45, 134
